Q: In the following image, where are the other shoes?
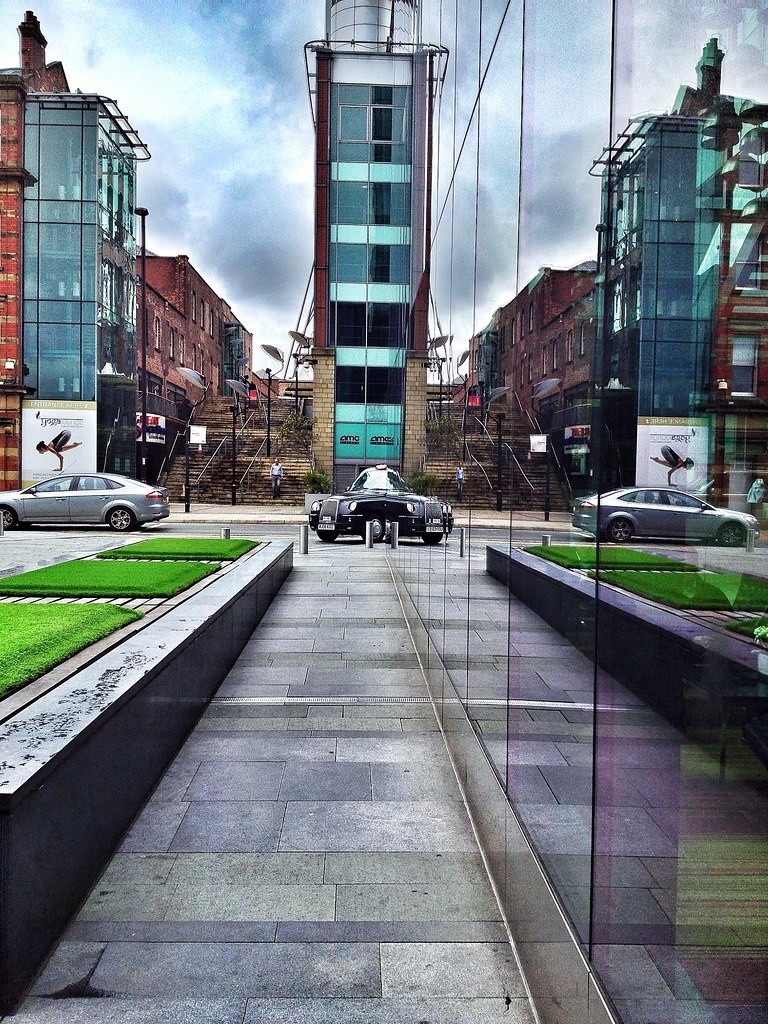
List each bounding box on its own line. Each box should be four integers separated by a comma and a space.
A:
277, 494, 280, 497
459, 501, 462, 503
273, 496, 276, 499
456, 498, 458, 501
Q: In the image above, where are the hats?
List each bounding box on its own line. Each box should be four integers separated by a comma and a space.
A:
274, 458, 279, 462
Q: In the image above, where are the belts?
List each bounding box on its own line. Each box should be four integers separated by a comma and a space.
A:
458, 478, 463, 480
274, 475, 280, 476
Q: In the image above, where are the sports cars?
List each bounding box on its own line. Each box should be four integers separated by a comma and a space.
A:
386, 462, 455, 546
307, 463, 386, 544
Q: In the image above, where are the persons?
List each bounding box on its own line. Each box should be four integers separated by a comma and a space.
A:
36, 430, 82, 471
650, 446, 695, 486
456, 461, 465, 502
270, 458, 284, 500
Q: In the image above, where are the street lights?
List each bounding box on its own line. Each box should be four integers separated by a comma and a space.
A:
132, 205, 150, 485
595, 221, 611, 276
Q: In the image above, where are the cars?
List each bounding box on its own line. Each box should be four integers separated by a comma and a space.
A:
0, 473, 172, 532
571, 485, 760, 547
